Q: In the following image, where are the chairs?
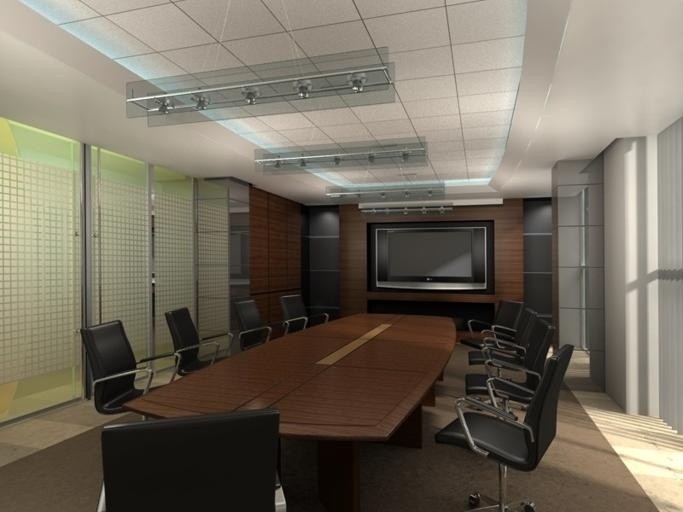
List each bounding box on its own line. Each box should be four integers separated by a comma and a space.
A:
96, 407, 289, 511
279, 294, 330, 336
232, 300, 290, 351
80, 320, 181, 421
165, 307, 233, 383
433, 297, 575, 511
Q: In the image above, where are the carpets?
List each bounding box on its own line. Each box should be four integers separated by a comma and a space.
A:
0, 376, 659, 512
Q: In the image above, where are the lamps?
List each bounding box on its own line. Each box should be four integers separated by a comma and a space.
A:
360, 204, 455, 217
125, 46, 395, 127
325, 182, 444, 202
254, 136, 428, 176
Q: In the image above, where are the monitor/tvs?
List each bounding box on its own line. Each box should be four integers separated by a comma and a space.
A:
375, 227, 488, 290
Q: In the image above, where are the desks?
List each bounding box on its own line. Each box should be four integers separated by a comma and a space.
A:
123, 312, 457, 512
